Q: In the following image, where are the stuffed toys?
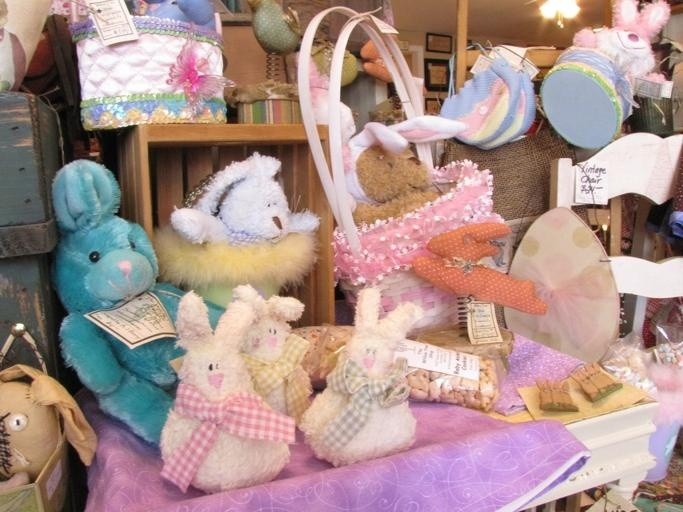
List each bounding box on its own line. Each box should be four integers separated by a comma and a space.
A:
159, 290, 296, 492
348, 116, 467, 227
134, 1, 215, 28
0, 1, 682, 512
157, 152, 321, 307
0, 381, 58, 481
52, 161, 223, 444
295, 52, 356, 146
573, 2, 671, 87
300, 288, 424, 467
234, 282, 313, 423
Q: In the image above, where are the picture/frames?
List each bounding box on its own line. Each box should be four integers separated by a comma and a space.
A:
424, 31, 452, 115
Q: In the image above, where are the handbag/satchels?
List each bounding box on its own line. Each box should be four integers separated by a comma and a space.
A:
330, 159, 511, 331
439, 40, 537, 151
69, 17, 231, 129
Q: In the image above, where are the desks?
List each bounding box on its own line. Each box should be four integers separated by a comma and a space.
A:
79, 326, 660, 512
